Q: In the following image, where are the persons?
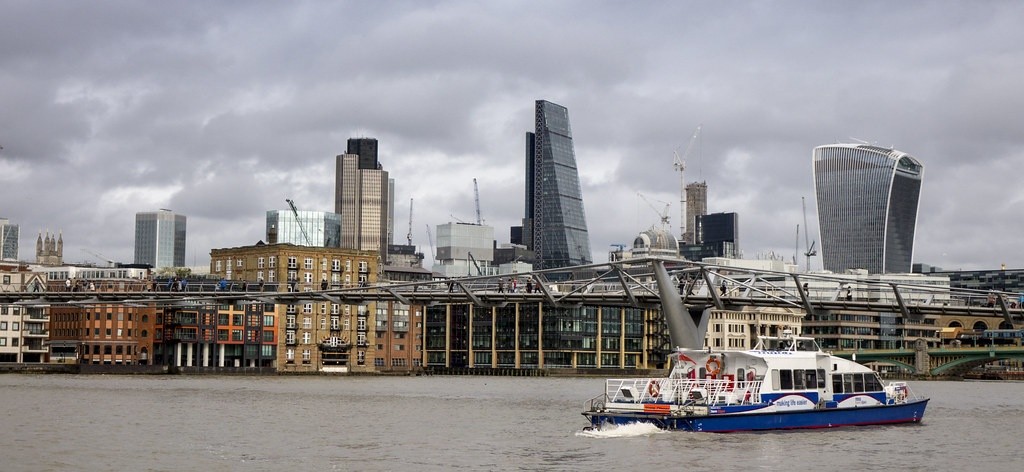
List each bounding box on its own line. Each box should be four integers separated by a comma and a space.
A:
678, 277, 696, 295
66, 277, 95, 292
497, 276, 517, 293
238, 278, 247, 292
145, 279, 156, 292
719, 280, 727, 297
291, 278, 299, 292
215, 277, 227, 292
361, 280, 371, 292
168, 273, 187, 292
259, 279, 264, 292
526, 277, 542, 293
987, 289, 995, 308
446, 277, 455, 292
804, 283, 810, 297
321, 279, 328, 290
413, 278, 419, 292
847, 286, 853, 301
33, 278, 40, 292
1017, 294, 1024, 309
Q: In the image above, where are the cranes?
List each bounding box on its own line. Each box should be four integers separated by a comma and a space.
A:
635, 192, 672, 228
801, 196, 817, 271
673, 124, 702, 240
406, 196, 413, 247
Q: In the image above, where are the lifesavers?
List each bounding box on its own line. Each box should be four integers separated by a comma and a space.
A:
649, 381, 660, 398
706, 358, 721, 374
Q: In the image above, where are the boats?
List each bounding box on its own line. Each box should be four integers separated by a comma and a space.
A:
582, 329, 931, 435
